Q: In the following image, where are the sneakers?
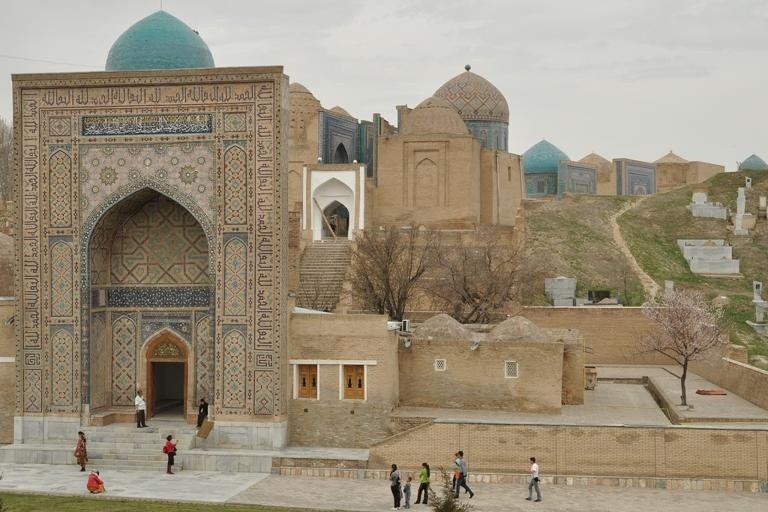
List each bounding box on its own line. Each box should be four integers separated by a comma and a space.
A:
403, 504, 410, 509
391, 506, 401, 511
81, 467, 86, 471
137, 425, 149, 428
414, 502, 428, 505
451, 489, 474, 499
525, 498, 543, 502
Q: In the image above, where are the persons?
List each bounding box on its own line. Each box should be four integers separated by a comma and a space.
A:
165, 435, 178, 474
451, 452, 467, 494
135, 390, 148, 428
194, 399, 209, 427
524, 457, 541, 501
403, 477, 412, 509
330, 215, 336, 232
74, 432, 88, 471
453, 450, 474, 499
87, 470, 104, 493
413, 462, 431, 504
391, 463, 402, 509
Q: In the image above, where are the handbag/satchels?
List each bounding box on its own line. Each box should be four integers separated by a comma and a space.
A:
73, 446, 82, 457
455, 464, 461, 481
534, 477, 538, 482
163, 447, 168, 454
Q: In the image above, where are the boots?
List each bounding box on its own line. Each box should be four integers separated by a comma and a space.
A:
166, 464, 174, 475
